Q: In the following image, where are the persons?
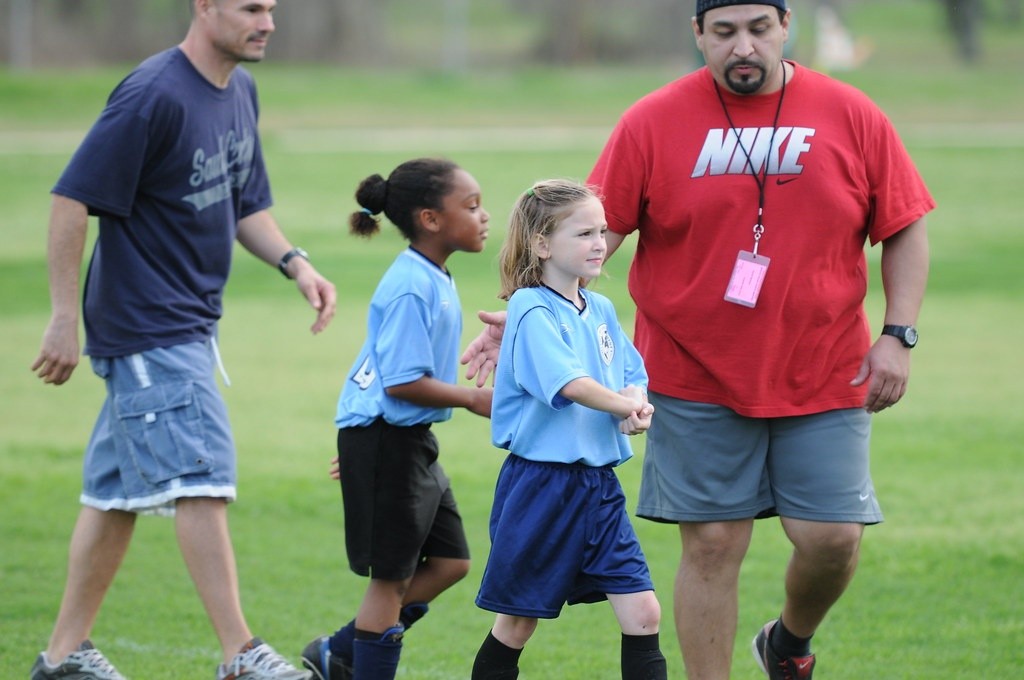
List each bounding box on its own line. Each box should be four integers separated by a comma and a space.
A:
460, 0, 935, 680
28, 0, 336, 678
301, 159, 495, 680
471, 178, 668, 679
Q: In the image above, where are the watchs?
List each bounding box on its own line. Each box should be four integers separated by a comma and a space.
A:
278, 247, 309, 280
881, 324, 919, 348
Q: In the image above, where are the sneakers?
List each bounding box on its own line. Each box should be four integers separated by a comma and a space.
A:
752, 621, 817, 680
217, 638, 314, 680
29, 640, 127, 680
303, 638, 359, 680
